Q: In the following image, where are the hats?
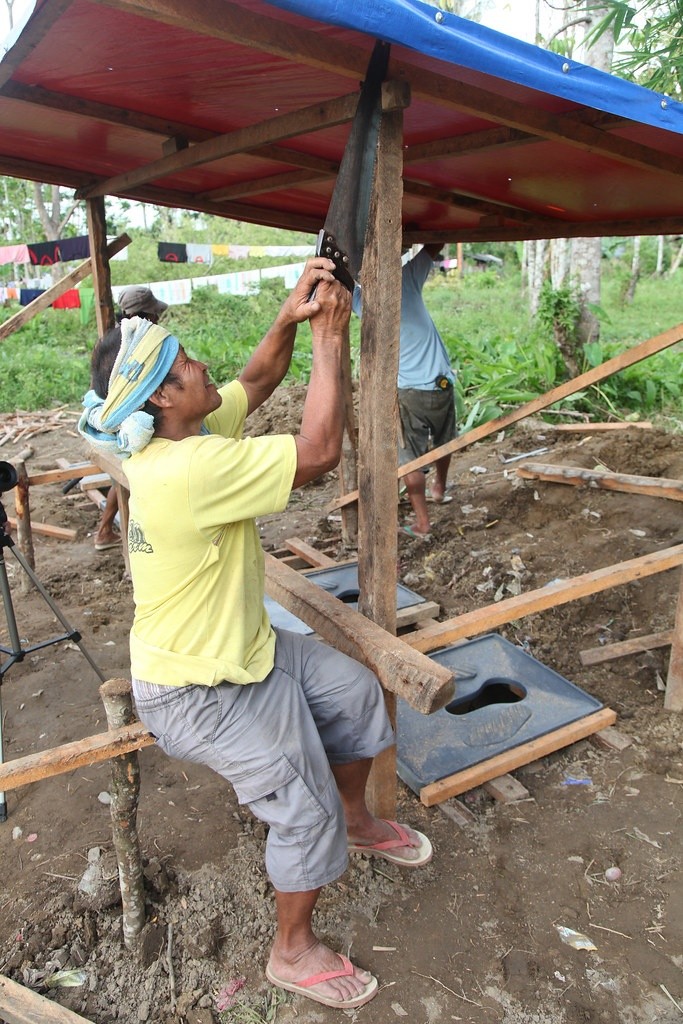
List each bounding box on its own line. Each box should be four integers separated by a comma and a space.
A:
118, 285, 168, 319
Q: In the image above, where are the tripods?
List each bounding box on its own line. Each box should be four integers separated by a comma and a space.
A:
0, 532, 106, 686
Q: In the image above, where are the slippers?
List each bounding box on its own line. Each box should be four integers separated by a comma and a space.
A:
398, 525, 418, 539
95, 537, 123, 550
424, 487, 432, 499
264, 950, 378, 1008
348, 818, 433, 866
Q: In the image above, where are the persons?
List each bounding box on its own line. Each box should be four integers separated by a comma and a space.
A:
77, 255, 433, 1009
349, 240, 456, 544
